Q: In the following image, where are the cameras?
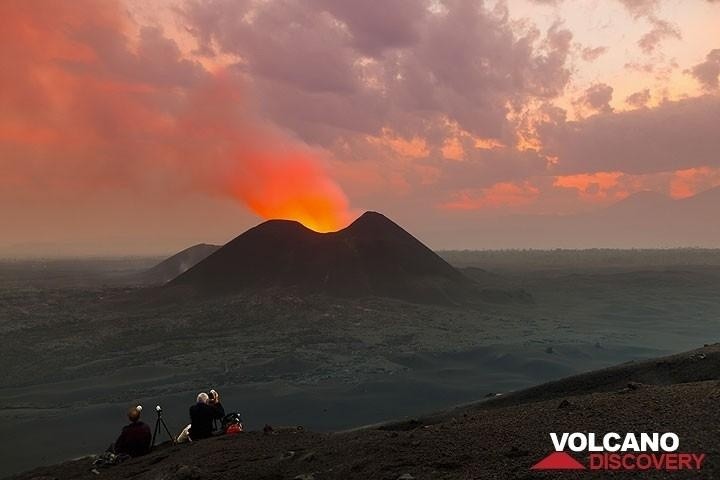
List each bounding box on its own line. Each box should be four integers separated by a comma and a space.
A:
208, 392, 219, 399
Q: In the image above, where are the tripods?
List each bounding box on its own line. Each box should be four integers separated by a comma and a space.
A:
151, 412, 174, 450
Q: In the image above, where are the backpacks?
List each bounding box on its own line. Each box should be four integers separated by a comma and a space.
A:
222, 412, 245, 434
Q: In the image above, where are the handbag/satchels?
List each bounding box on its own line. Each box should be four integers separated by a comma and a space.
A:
101, 452, 120, 464
176, 423, 192, 443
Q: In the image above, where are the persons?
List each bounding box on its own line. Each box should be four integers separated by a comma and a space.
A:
173, 390, 225, 445
105, 407, 151, 459
